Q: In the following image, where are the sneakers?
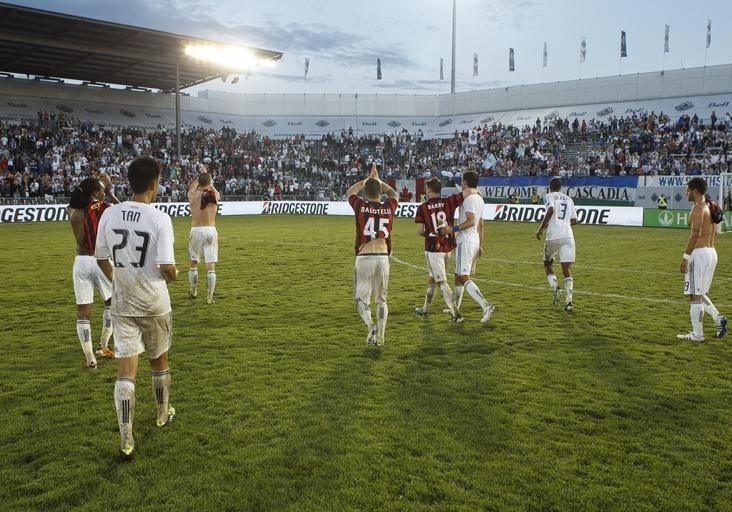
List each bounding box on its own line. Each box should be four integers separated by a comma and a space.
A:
677, 332, 705, 342
716, 313, 727, 338
366, 284, 572, 347
83, 290, 215, 464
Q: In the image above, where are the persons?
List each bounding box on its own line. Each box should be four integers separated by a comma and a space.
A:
414, 177, 482, 323
186, 162, 221, 305
95, 156, 179, 459
67, 171, 123, 367
345, 163, 400, 346
455, 109, 732, 209
1, 109, 191, 205
537, 178, 579, 312
188, 122, 457, 201
677, 177, 728, 342
443, 171, 498, 321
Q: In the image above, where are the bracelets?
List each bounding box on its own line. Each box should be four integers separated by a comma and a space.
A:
683, 254, 691, 261
379, 178, 382, 183
364, 176, 368, 185
454, 225, 459, 231
